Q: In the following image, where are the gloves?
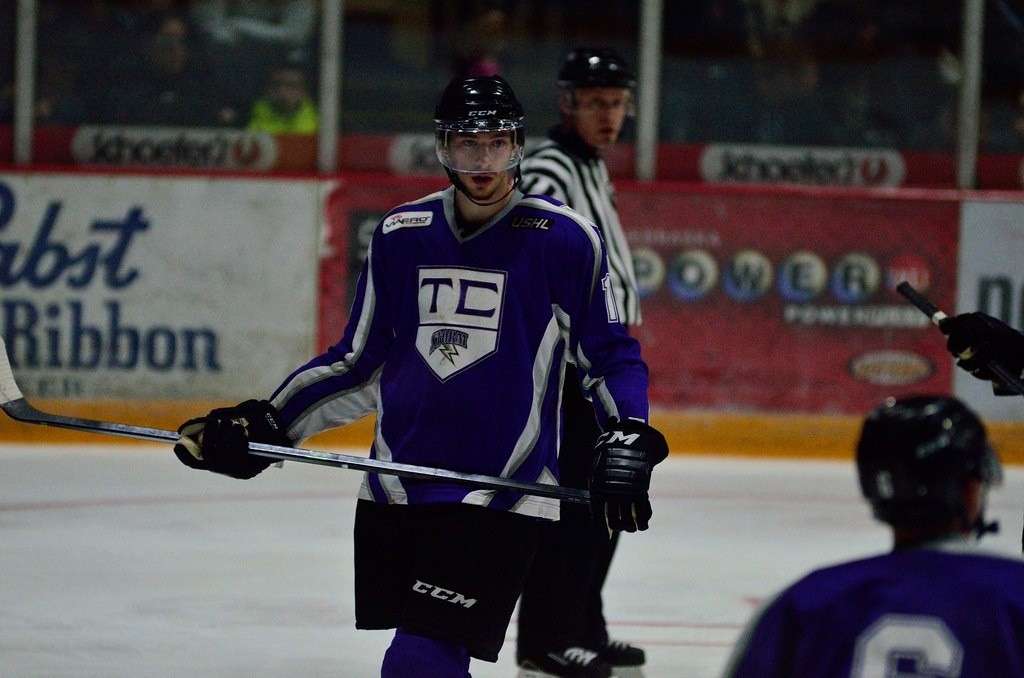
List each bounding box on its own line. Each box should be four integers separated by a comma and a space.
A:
592, 419, 669, 532
178, 400, 292, 481
940, 312, 1023, 396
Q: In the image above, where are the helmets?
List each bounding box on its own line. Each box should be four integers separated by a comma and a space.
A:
854, 394, 999, 540
557, 47, 632, 89
435, 72, 527, 191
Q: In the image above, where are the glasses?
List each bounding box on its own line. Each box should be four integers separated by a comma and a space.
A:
271, 79, 294, 88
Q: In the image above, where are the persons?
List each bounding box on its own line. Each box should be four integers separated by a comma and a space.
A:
722, 392, 1024, 678
0, 0, 1023, 157
174, 75, 671, 678
939, 280, 1024, 397
519, 44, 646, 678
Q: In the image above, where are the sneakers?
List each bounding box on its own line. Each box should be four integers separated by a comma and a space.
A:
517, 640, 645, 678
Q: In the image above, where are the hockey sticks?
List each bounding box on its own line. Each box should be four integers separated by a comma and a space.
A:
1, 334, 559, 497
895, 276, 972, 359
